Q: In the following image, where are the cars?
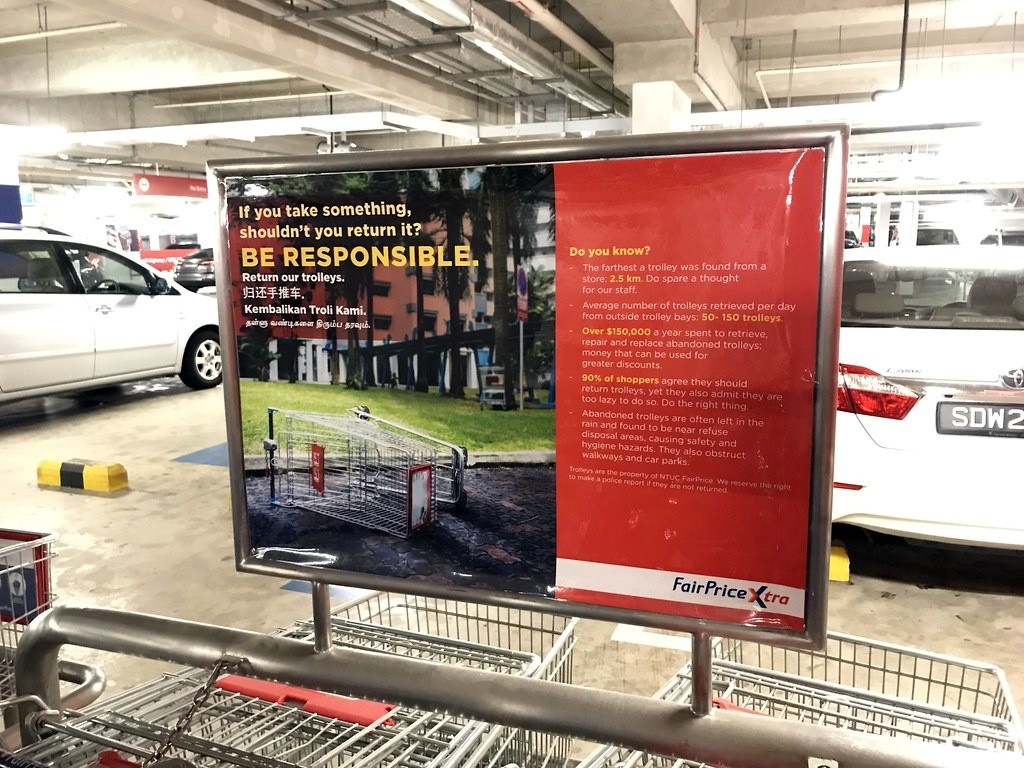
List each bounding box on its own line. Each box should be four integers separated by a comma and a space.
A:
977, 230, 1024, 250
844, 231, 862, 248
172, 246, 216, 294
0, 223, 223, 406
479, 383, 506, 411
832, 246, 1024, 555
916, 223, 960, 248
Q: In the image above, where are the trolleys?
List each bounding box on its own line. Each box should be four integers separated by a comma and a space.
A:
261, 404, 471, 540
1, 527, 1023, 768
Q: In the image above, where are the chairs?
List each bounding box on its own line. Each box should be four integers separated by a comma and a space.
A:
23, 258, 65, 291
841, 271, 1024, 328
